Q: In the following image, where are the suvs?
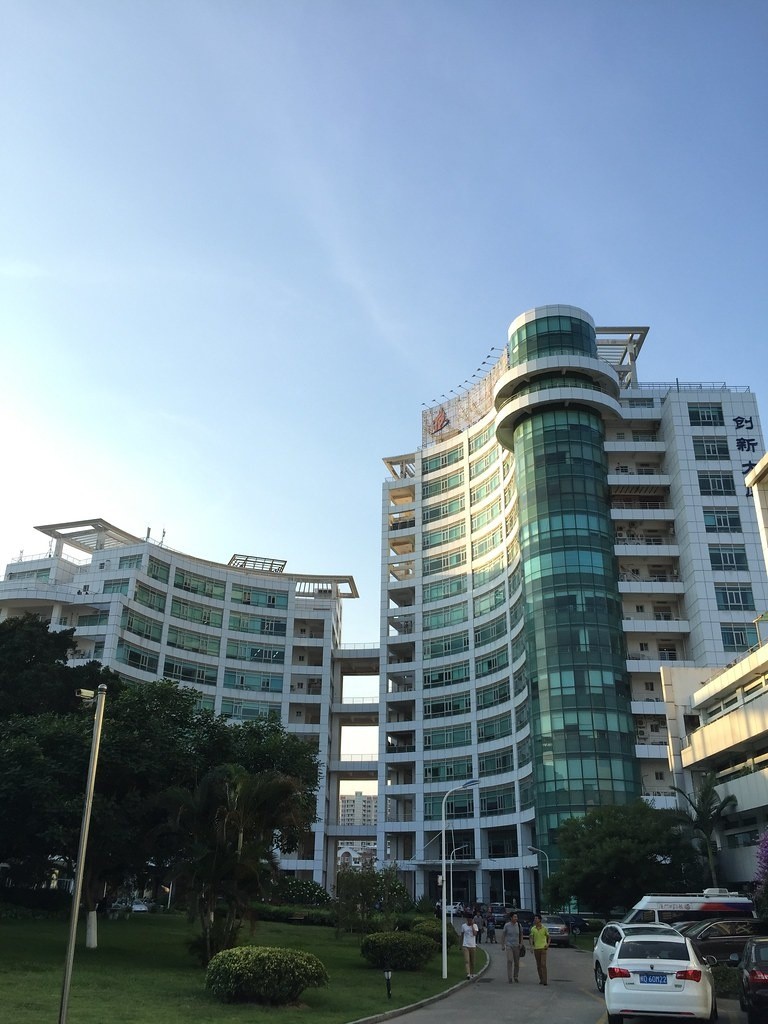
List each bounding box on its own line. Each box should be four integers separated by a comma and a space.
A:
593, 921, 685, 993
682, 917, 767, 967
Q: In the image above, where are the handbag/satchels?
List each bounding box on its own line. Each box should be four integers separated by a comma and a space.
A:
519, 945, 526, 957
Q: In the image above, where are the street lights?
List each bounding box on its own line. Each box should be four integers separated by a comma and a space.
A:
526, 845, 549, 878
450, 844, 468, 927
441, 779, 480, 979
58, 681, 109, 1024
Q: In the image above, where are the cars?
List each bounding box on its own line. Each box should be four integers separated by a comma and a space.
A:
529, 914, 569, 948
445, 901, 465, 917
503, 909, 535, 936
603, 933, 720, 1024
670, 919, 699, 934
112, 897, 148, 912
730, 936, 768, 1024
482, 906, 507, 929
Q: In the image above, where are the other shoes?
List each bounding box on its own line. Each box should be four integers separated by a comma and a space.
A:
509, 979, 512, 984
470, 974, 474, 979
514, 976, 518, 983
467, 975, 470, 980
539, 982, 542, 984
544, 983, 547, 986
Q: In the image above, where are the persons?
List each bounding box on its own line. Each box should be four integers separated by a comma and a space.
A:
434, 899, 497, 944
500, 912, 523, 984
528, 915, 551, 986
459, 914, 479, 978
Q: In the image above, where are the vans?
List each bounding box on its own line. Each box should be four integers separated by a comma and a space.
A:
558, 914, 591, 935
622, 887, 759, 931
490, 902, 523, 915
